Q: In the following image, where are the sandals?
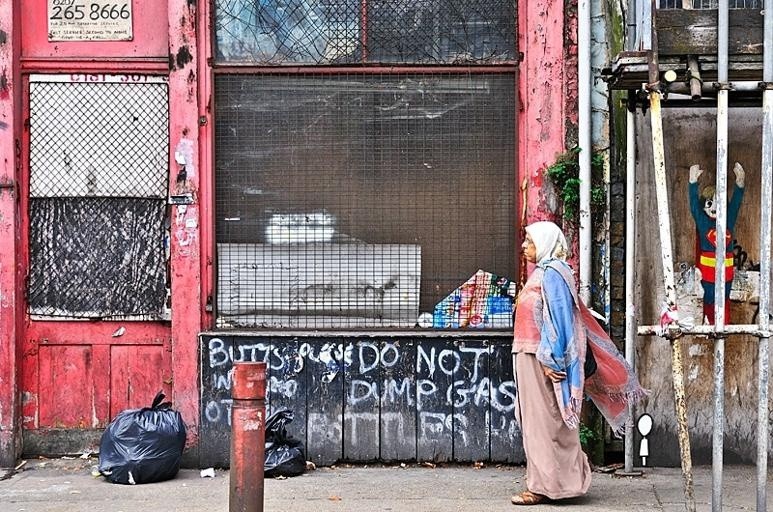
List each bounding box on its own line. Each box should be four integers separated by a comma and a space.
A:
511, 491, 545, 504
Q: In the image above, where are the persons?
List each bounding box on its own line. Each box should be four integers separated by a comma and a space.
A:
511, 220, 651, 505
689, 161, 746, 326
